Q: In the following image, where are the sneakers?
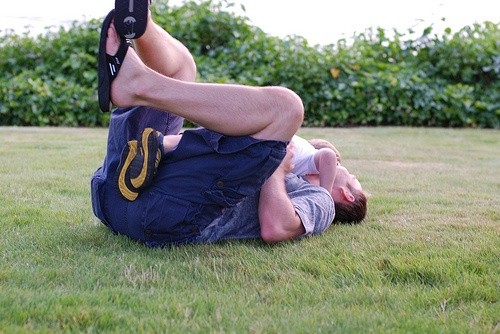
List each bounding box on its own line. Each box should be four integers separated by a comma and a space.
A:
115, 127, 164, 202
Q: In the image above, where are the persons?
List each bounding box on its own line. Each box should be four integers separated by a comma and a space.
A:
90, 0, 367, 247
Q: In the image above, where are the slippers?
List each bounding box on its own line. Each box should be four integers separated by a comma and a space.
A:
115, 0, 149, 37
98, 9, 135, 112
